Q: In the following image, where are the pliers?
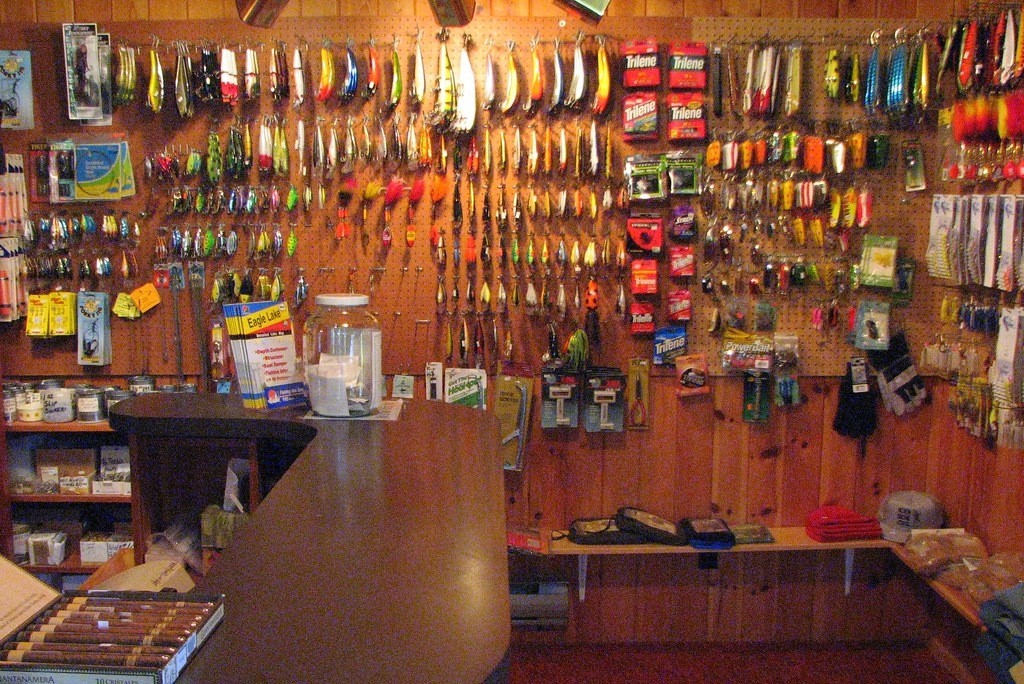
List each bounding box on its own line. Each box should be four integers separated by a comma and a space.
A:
629, 373, 647, 426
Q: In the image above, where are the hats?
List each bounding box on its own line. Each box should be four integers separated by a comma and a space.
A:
877, 490, 947, 543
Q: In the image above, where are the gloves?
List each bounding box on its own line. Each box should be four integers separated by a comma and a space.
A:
867, 330, 927, 417
832, 362, 883, 440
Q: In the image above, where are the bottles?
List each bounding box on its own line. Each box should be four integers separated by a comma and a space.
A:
301, 293, 384, 418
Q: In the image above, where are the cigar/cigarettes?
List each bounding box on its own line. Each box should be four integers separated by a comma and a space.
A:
0, 593, 213, 668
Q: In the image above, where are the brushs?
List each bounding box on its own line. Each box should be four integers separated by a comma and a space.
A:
926, 224, 1024, 291
992, 375, 1024, 403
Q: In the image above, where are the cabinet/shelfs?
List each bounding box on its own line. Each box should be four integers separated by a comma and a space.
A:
0, 420, 132, 575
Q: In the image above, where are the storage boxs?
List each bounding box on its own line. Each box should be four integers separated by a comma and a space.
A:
91, 471, 132, 496
35, 447, 96, 484
0, 556, 225, 684
79, 534, 108, 569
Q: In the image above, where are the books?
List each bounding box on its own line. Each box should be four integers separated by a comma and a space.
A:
224, 299, 308, 411
